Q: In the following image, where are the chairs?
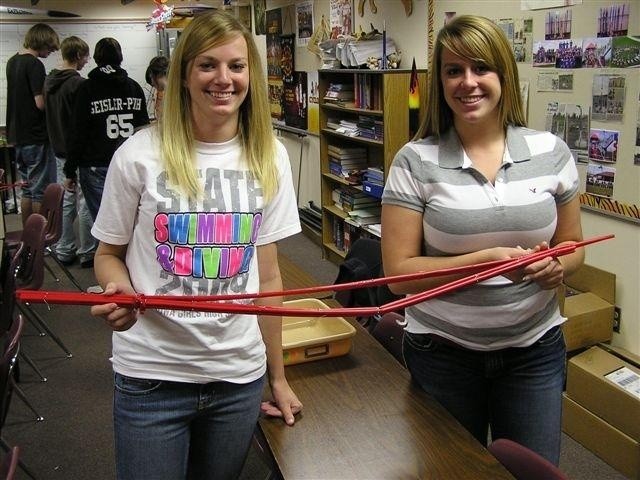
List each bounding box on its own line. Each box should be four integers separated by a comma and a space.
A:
488, 439, 568, 479
370, 314, 410, 364
1, 182, 88, 480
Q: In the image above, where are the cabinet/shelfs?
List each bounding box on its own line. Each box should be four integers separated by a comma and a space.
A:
318, 67, 429, 270
557, 262, 640, 480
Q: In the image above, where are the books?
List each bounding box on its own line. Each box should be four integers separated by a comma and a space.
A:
323, 81, 384, 256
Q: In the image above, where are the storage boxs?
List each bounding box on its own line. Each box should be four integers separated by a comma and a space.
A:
274, 297, 355, 366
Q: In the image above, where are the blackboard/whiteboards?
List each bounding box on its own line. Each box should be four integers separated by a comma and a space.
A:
0, 19, 164, 127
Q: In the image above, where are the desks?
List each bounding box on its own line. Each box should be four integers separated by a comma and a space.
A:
255, 299, 517, 479
273, 254, 337, 303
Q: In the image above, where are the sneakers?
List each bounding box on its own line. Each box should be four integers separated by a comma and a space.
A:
60, 260, 94, 268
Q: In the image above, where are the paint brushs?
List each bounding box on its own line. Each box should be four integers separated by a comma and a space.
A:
0, 6, 81, 17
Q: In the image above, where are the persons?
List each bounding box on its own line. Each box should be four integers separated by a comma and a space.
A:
568, 105, 584, 147
145, 55, 171, 94
84, 10, 305, 480
533, 40, 583, 69
61, 37, 152, 224
43, 35, 98, 268
5, 22, 59, 232
380, 12, 587, 471
